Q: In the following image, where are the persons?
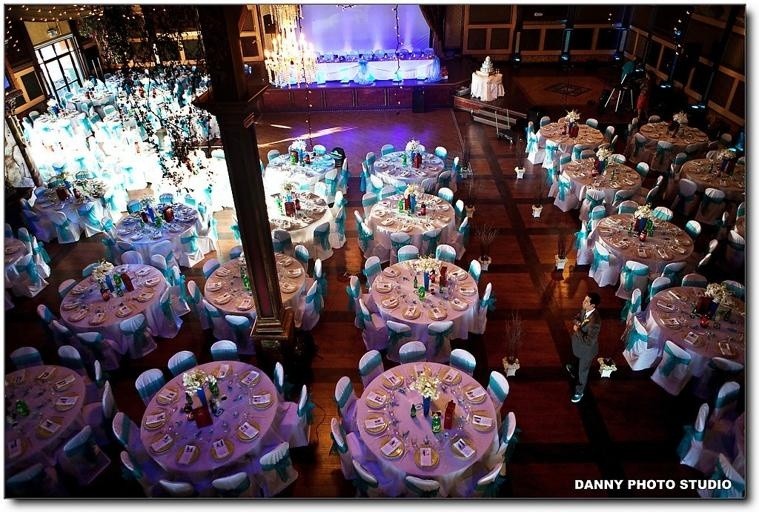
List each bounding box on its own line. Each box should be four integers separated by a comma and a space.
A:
566, 292, 602, 403
636, 70, 657, 121
5, 180, 39, 243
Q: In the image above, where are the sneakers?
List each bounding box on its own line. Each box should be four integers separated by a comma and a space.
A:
570, 390, 584, 403
566, 364, 576, 379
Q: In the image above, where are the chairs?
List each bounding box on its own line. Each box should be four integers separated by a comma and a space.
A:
6, 136, 531, 500
521, 110, 748, 499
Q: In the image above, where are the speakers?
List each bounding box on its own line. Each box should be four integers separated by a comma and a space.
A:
263, 14, 278, 35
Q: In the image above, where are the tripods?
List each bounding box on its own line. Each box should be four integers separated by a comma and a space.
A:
603, 70, 635, 113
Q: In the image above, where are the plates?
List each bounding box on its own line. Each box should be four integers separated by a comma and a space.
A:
539, 122, 746, 358
5, 152, 496, 472
